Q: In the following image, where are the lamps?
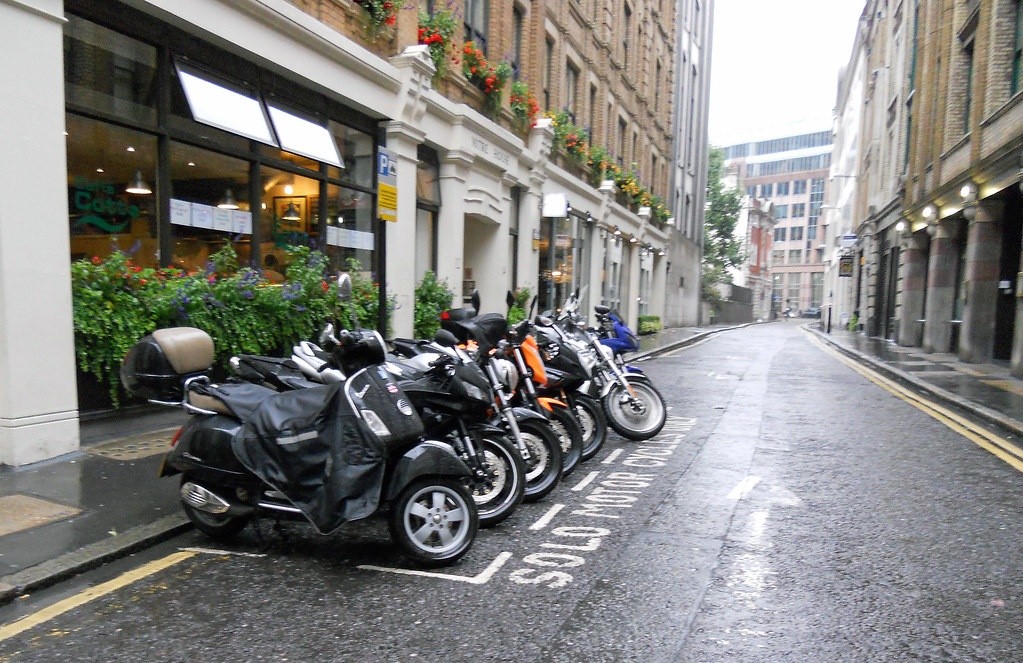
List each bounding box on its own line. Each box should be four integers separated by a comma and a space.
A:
565, 213, 570, 221
657, 247, 665, 255
960, 181, 979, 203
566, 201, 572, 211
646, 242, 653, 250
216, 157, 239, 210
125, 131, 152, 195
585, 211, 592, 223
629, 233, 637, 243
895, 219, 912, 235
922, 203, 940, 221
612, 225, 621, 235
610, 234, 616, 242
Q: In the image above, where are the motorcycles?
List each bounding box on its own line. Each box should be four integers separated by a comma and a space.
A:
122, 287, 667, 570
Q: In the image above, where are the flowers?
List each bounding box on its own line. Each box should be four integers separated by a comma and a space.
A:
359, 0, 621, 183
72, 242, 456, 349
618, 172, 672, 225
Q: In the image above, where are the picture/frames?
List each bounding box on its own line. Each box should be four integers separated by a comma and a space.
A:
272, 195, 307, 236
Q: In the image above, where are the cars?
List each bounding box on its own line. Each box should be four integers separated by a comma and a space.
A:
801, 308, 821, 319
